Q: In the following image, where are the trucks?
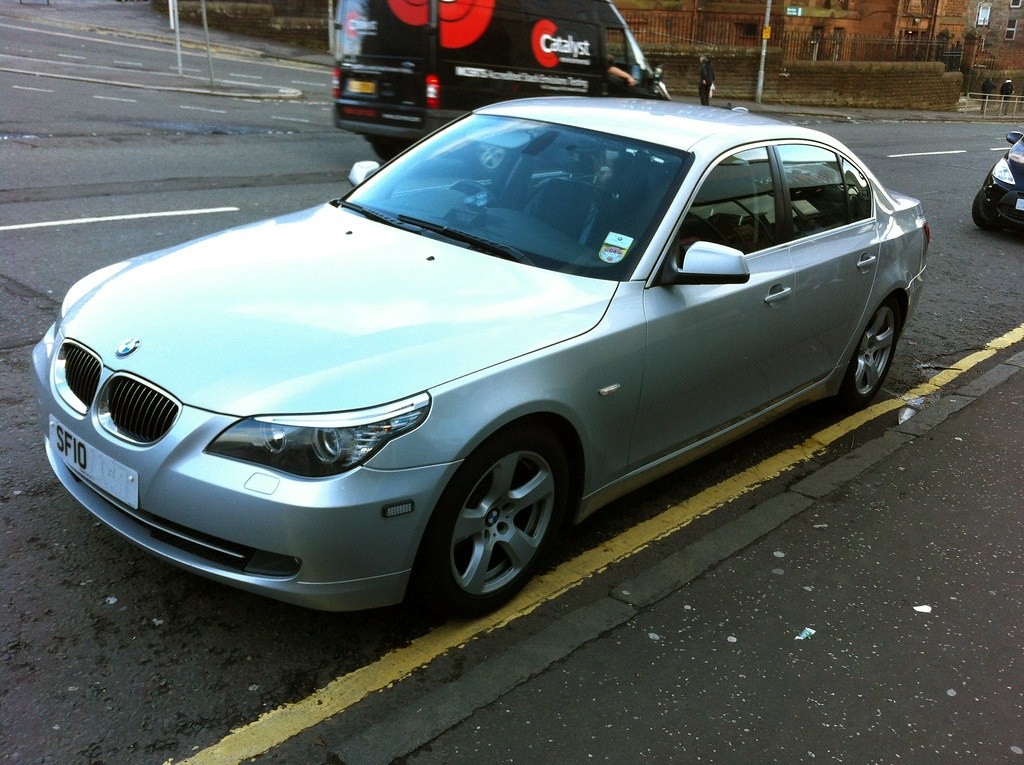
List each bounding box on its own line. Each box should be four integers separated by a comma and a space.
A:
332, 2, 669, 160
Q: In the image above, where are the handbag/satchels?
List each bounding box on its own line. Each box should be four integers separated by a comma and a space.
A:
709, 82, 716, 97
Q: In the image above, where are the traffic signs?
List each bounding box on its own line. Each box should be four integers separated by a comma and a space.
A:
783, 3, 804, 23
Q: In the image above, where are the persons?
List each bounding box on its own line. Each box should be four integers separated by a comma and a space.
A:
698, 53, 716, 106
999, 76, 1014, 116
980, 75, 995, 113
608, 53, 636, 86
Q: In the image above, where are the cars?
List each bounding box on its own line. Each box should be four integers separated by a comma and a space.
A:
32, 97, 932, 611
970, 125, 1024, 236
